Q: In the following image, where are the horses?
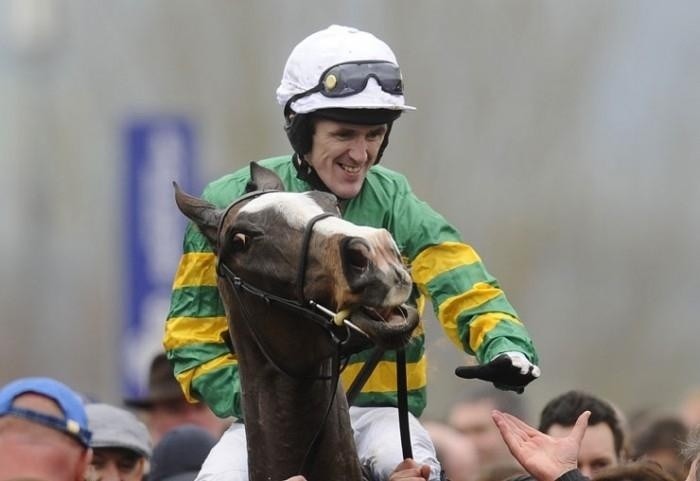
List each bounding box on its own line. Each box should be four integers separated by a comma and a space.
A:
171, 158, 421, 480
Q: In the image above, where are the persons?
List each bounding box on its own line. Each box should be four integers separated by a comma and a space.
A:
0, 354, 249, 480
388, 388, 700, 481
161, 23, 542, 481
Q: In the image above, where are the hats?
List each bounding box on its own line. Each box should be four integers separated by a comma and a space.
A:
0, 377, 91, 446
85, 403, 154, 460
148, 425, 219, 481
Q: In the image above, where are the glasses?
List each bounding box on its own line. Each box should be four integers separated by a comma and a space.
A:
318, 60, 404, 111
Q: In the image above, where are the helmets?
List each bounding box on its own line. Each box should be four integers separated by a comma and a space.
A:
276, 23, 418, 115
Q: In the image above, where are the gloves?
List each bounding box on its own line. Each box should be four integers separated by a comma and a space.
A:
455, 351, 541, 394
